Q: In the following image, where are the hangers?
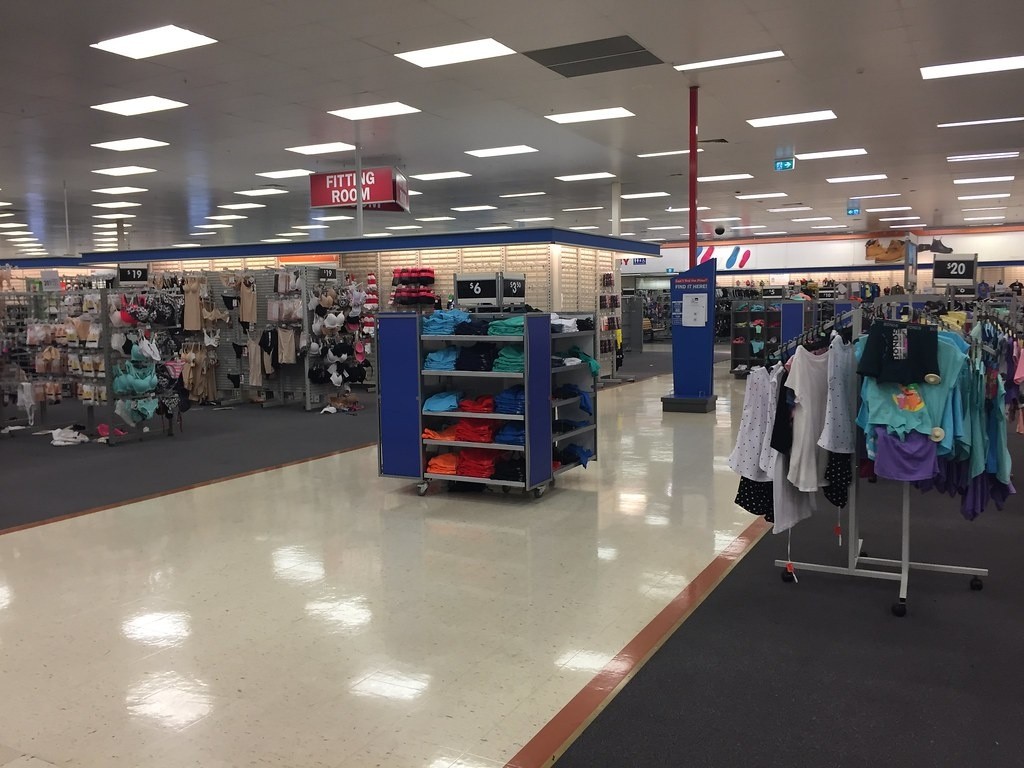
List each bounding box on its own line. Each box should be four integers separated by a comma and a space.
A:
741, 305, 1024, 376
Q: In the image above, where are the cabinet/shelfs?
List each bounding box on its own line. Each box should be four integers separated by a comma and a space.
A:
621, 281, 945, 379
374, 311, 597, 497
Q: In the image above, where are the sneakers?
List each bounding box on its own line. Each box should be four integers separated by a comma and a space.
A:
916, 236, 954, 254
865, 239, 906, 264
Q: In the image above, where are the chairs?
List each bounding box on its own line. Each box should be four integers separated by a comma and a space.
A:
643, 318, 665, 343
